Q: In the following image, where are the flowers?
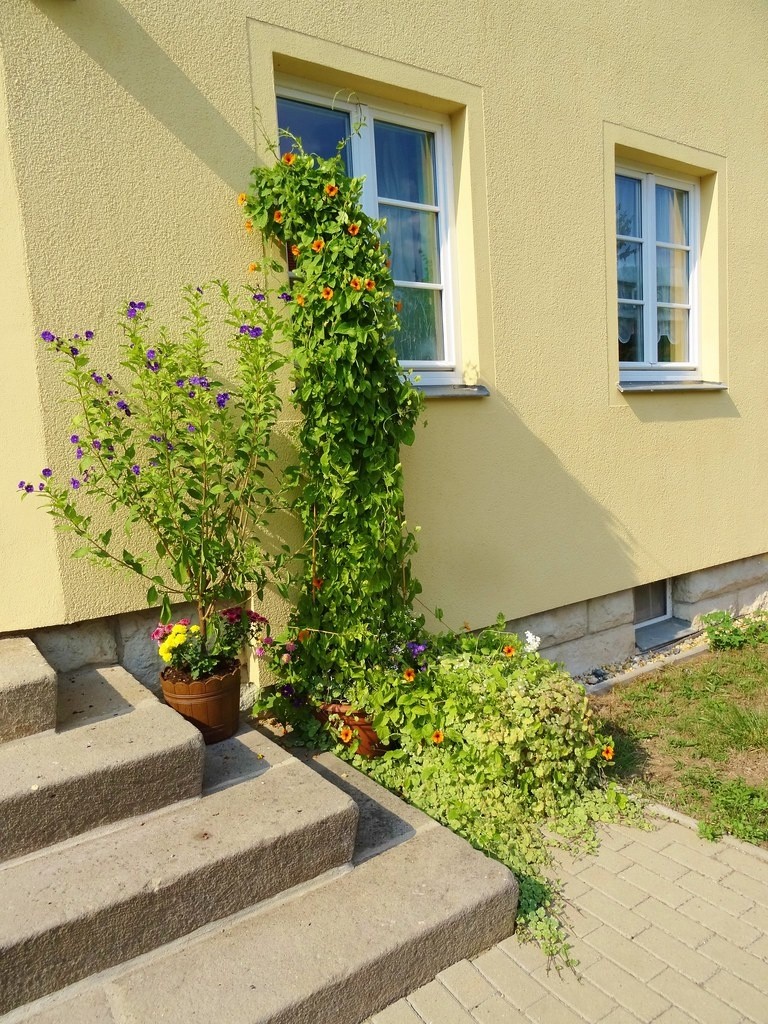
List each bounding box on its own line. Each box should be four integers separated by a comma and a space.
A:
15, 278, 345, 682
238, 84, 533, 766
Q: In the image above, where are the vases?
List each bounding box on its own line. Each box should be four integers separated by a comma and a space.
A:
307, 694, 386, 758
159, 657, 241, 745
